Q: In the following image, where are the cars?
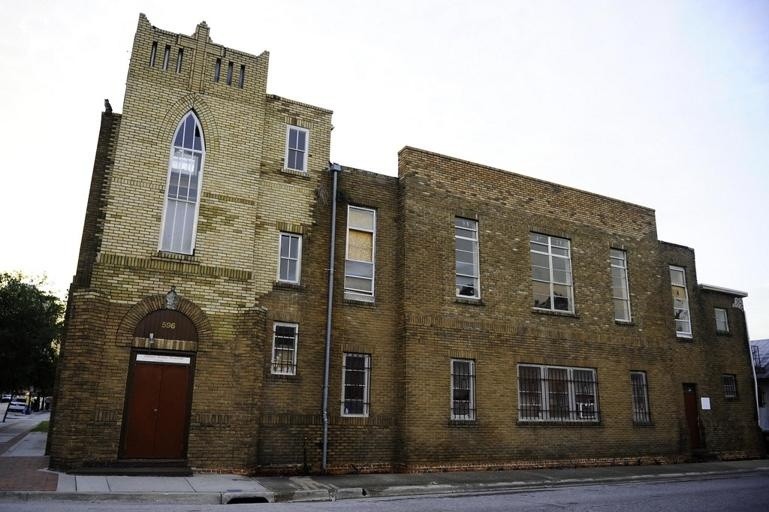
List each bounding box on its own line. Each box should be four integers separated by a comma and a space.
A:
2, 395, 44, 413
